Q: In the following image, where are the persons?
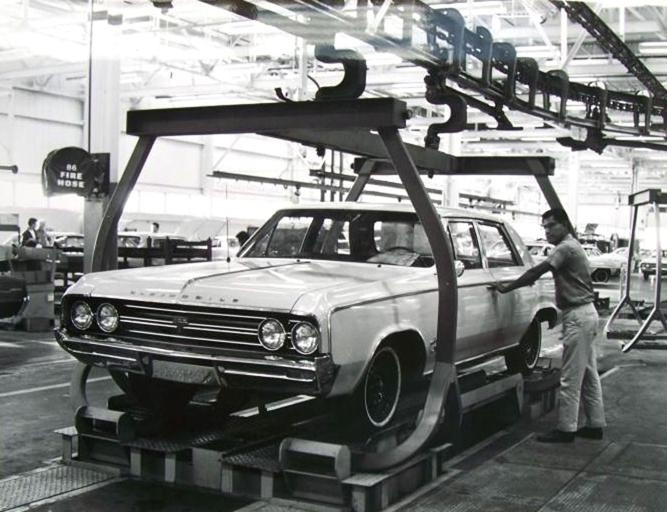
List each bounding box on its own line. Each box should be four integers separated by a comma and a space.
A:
21, 217, 38, 248
35, 219, 52, 247
151, 222, 159, 233
489, 209, 608, 445
234, 231, 248, 247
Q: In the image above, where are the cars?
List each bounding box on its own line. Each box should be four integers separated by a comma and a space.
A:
0, 232, 240, 315
526, 232, 667, 282
52, 203, 561, 438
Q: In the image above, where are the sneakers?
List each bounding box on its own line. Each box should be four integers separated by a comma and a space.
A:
535, 426, 605, 443
